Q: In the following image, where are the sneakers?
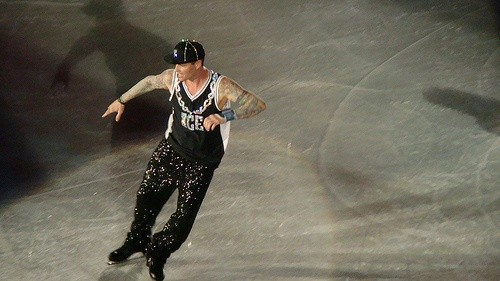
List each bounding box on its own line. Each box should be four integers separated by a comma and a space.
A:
144, 243, 170, 281
107, 243, 144, 266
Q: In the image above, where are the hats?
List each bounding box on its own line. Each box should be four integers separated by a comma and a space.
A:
163, 39, 206, 64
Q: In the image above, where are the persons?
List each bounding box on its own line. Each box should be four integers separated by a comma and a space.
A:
100, 38, 271, 280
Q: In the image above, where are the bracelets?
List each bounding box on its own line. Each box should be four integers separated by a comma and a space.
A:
118, 97, 126, 105
